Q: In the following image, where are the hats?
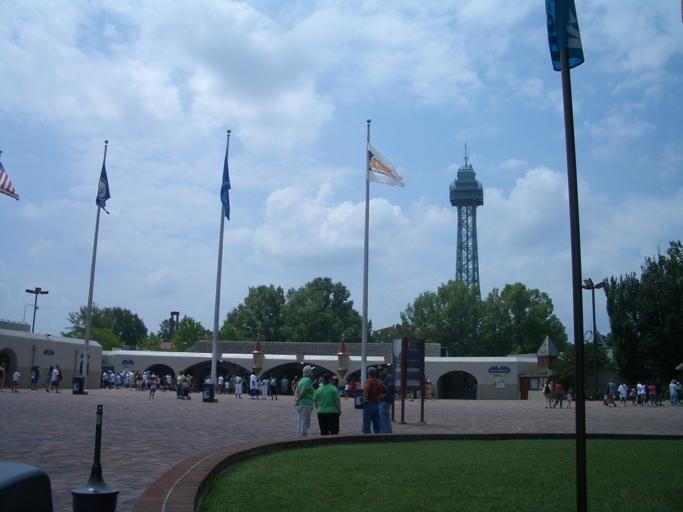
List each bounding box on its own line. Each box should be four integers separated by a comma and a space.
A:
303, 365, 316, 373
368, 367, 377, 376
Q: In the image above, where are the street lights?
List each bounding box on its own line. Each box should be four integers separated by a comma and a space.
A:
23, 286, 48, 333
581, 278, 607, 399
22, 303, 39, 320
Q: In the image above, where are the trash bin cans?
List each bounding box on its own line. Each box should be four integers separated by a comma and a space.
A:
354, 389, 364, 409
72, 376, 85, 394
202, 383, 215, 402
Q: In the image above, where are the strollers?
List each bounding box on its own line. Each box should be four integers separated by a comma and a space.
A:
180, 382, 192, 400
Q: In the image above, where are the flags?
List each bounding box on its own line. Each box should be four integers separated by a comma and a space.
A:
220, 141, 231, 220
0, 160, 18, 200
366, 141, 405, 188
96, 157, 110, 215
544, 1, 585, 71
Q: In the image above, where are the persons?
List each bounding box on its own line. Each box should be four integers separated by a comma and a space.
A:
604, 378, 681, 407
361, 366, 387, 432
12, 368, 21, 393
313, 372, 342, 435
407, 377, 434, 402
541, 379, 565, 408
102, 368, 156, 391
175, 371, 186, 400
31, 365, 40, 391
344, 378, 351, 400
1, 364, 7, 392
376, 364, 395, 433
563, 390, 573, 407
42, 364, 62, 393
294, 364, 315, 437
242, 376, 297, 395
351, 377, 357, 397
157, 372, 194, 392
257, 376, 277, 400
148, 378, 157, 399
194, 374, 236, 394
247, 368, 257, 399
234, 372, 244, 399
